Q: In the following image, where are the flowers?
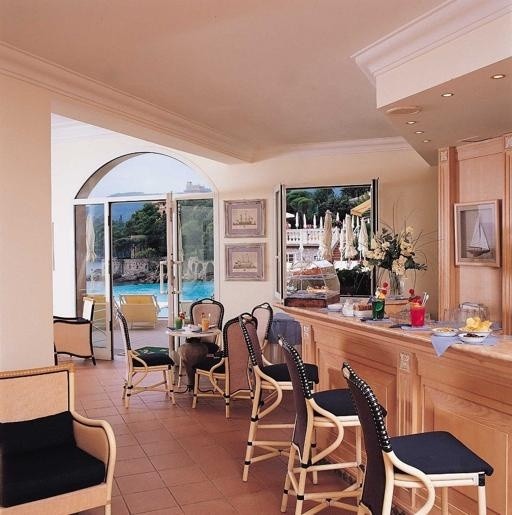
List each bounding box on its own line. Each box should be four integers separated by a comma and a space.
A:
363, 202, 441, 292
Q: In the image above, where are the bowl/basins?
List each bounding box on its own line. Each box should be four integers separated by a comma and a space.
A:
354, 310, 373, 317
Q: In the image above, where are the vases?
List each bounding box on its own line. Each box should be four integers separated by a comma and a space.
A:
387, 275, 407, 299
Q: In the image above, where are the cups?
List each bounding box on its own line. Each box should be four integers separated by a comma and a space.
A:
202, 314, 210, 332
371, 298, 385, 320
405, 307, 426, 328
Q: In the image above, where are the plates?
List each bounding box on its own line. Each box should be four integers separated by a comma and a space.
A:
459, 334, 487, 342
460, 328, 492, 336
401, 325, 430, 330
432, 327, 458, 336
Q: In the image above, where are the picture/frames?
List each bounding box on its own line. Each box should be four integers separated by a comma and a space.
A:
224, 242, 266, 282
454, 200, 501, 269
223, 198, 268, 238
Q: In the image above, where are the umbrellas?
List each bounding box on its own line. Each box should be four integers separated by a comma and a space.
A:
313, 214, 316, 229
358, 217, 368, 260
294, 211, 300, 229
86, 214, 97, 289
319, 210, 332, 262
302, 214, 307, 228
345, 214, 357, 262
320, 216, 323, 229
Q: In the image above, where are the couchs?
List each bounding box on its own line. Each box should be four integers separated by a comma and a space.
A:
0, 363, 117, 515
52, 297, 96, 366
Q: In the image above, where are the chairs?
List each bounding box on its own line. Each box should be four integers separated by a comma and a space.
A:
341, 361, 494, 515
216, 302, 273, 401
240, 312, 320, 484
193, 314, 258, 419
190, 298, 224, 391
115, 307, 175, 408
277, 335, 387, 514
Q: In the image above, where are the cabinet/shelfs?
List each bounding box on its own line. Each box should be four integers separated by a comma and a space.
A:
273, 302, 512, 515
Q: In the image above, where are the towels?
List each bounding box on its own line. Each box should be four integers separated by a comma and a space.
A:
432, 328, 496, 358
